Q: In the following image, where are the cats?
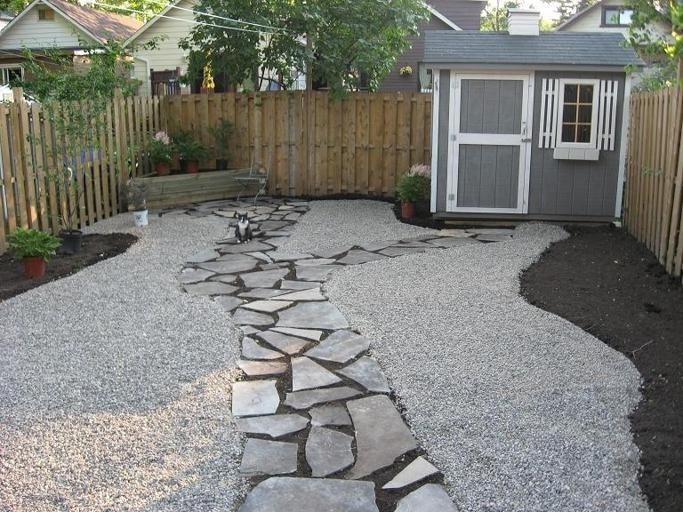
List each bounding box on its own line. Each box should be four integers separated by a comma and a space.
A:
235, 212, 253, 244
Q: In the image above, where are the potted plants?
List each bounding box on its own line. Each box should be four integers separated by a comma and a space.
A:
4, 226, 65, 279
144, 117, 236, 176
23, 132, 110, 251
394, 175, 431, 218
117, 179, 152, 228
